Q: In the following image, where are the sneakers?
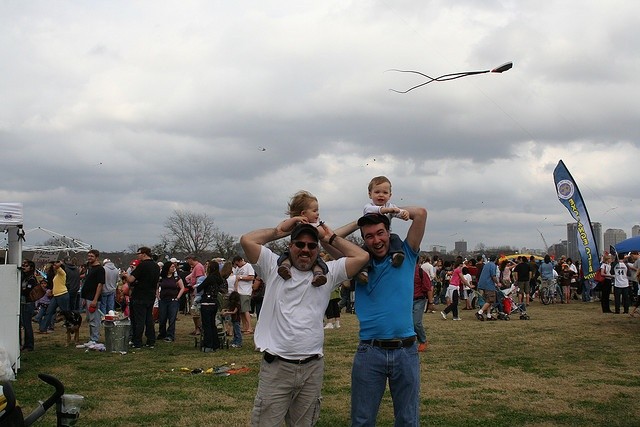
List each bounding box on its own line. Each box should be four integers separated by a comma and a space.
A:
487, 316, 497, 321
311, 275, 327, 287
392, 255, 404, 268
324, 323, 333, 330
357, 273, 368, 285
475, 312, 484, 321
333, 322, 340, 329
452, 318, 461, 321
84, 341, 96, 347
277, 266, 291, 280
440, 311, 447, 320
418, 342, 427, 352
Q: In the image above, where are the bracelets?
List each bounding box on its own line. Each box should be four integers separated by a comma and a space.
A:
329, 234, 336, 245
240, 276, 243, 280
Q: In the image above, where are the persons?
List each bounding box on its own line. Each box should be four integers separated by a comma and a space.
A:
559, 265, 576, 304
421, 258, 437, 300
357, 176, 410, 284
320, 206, 427, 426
155, 261, 185, 343
100, 258, 119, 324
601, 254, 615, 313
514, 256, 532, 306
222, 290, 242, 348
152, 262, 164, 325
125, 248, 141, 345
500, 259, 516, 301
609, 253, 631, 314
627, 250, 640, 307
219, 262, 238, 336
190, 276, 206, 309
435, 259, 441, 304
468, 259, 482, 309
276, 190, 329, 287
233, 255, 255, 335
21, 260, 46, 352
552, 268, 564, 303
240, 221, 370, 427
32, 279, 55, 331
501, 288, 519, 313
566, 258, 578, 300
63, 255, 80, 310
413, 256, 433, 352
460, 267, 472, 310
195, 261, 220, 353
456, 256, 466, 272
40, 260, 72, 332
129, 260, 140, 273
167, 258, 185, 321
439, 261, 453, 304
475, 256, 485, 275
529, 256, 540, 302
440, 258, 475, 321
337, 279, 355, 314
556, 258, 566, 270
81, 249, 106, 349
253, 276, 265, 321
219, 257, 226, 264
475, 254, 502, 321
204, 260, 210, 269
184, 254, 205, 335
538, 254, 557, 304
121, 247, 160, 349
323, 254, 342, 330
442, 266, 453, 304
626, 256, 634, 306
494, 260, 501, 304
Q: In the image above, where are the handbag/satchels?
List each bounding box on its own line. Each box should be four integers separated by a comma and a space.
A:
594, 265, 606, 282
29, 277, 46, 303
503, 296, 512, 315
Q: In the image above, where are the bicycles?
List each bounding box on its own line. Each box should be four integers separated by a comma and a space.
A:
541, 275, 563, 304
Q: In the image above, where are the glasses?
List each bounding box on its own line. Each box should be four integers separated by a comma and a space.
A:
358, 214, 378, 226
294, 242, 318, 251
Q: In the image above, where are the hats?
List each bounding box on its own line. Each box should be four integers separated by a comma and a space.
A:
292, 224, 319, 239
170, 258, 180, 263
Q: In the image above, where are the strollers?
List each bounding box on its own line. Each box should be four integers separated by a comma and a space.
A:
194, 291, 229, 348
494, 283, 530, 320
470, 289, 500, 315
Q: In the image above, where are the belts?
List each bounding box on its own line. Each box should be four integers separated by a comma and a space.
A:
361, 337, 416, 349
276, 355, 318, 365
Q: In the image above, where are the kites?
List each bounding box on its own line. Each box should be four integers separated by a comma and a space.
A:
383, 60, 513, 93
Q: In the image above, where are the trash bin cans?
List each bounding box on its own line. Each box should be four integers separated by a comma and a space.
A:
102, 315, 134, 354
